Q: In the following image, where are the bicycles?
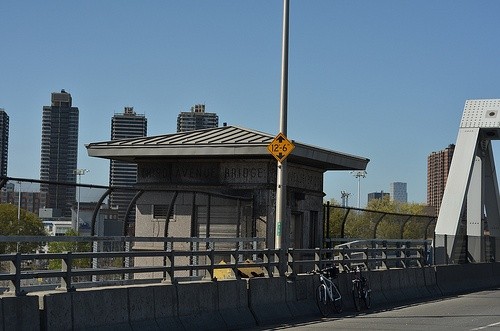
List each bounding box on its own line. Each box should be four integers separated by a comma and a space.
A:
345, 264, 371, 313
306, 266, 343, 318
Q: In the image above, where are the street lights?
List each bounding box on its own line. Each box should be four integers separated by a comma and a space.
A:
73, 168, 89, 232
350, 171, 367, 212
15, 177, 22, 222
